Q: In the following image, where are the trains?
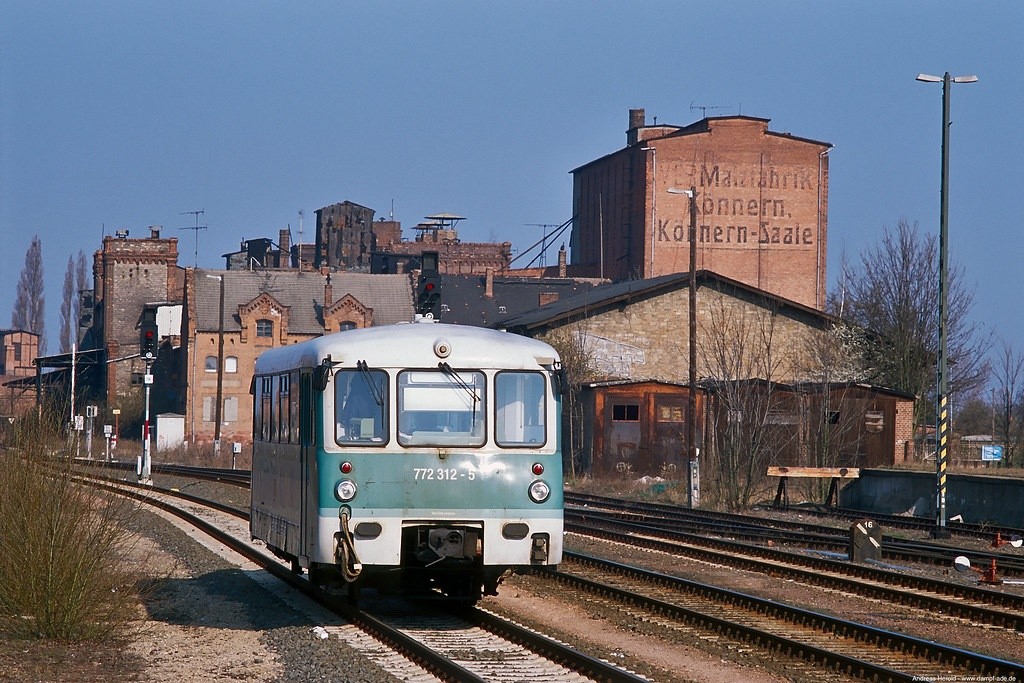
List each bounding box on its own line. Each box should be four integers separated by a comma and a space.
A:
245, 320, 563, 611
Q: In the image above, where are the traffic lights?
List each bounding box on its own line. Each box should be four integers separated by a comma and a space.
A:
146, 332, 155, 355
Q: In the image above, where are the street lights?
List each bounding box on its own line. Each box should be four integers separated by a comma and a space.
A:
640, 145, 658, 280
667, 187, 701, 510
915, 71, 978, 544
205, 273, 225, 452
